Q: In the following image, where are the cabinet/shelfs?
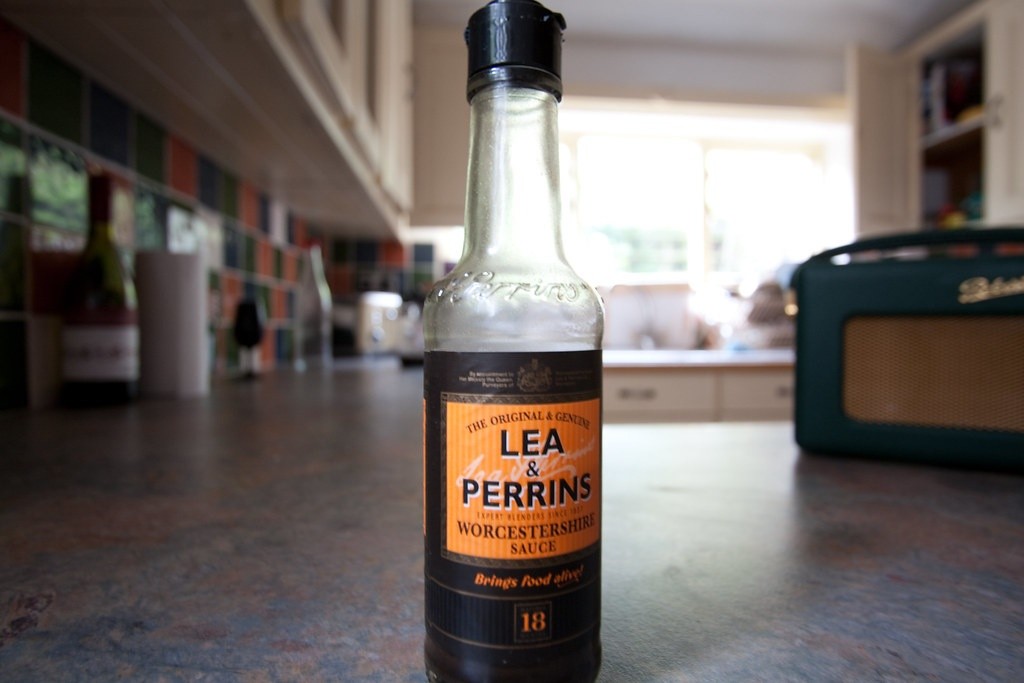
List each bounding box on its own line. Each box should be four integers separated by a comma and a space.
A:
13, 0, 413, 247
842, 0, 1024, 251
603, 349, 798, 420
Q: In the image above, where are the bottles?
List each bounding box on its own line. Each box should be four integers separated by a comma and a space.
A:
292, 236, 335, 373
422, 0, 606, 682
55, 169, 141, 405
